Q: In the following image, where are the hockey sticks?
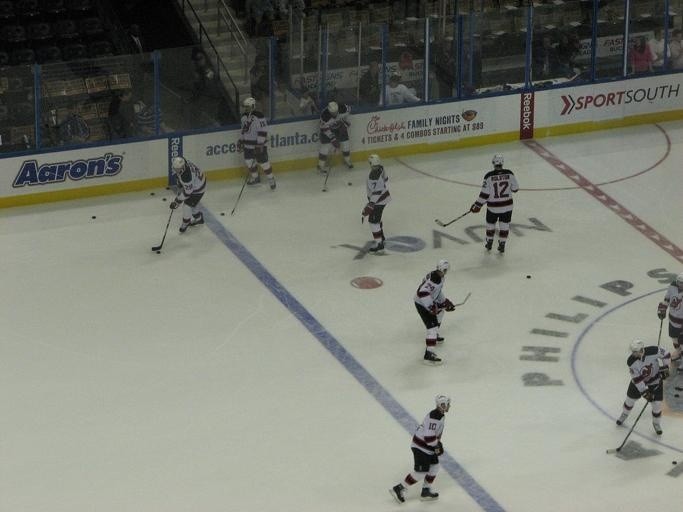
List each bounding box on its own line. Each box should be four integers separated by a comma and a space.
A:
152, 208, 174, 250
438, 292, 471, 311
231, 157, 256, 215
322, 148, 337, 187
435, 209, 471, 227
607, 400, 648, 454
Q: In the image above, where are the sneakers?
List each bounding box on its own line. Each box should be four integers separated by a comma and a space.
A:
436, 336, 444, 342
270, 181, 276, 190
370, 241, 384, 252
318, 165, 327, 175
179, 218, 203, 232
247, 178, 259, 184
344, 160, 353, 168
424, 352, 442, 361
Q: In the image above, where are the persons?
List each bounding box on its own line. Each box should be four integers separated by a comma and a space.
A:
657, 275, 683, 361
56, 0, 682, 189
361, 155, 392, 251
470, 154, 519, 252
414, 259, 455, 361
616, 336, 671, 434
169, 156, 206, 233
392, 394, 452, 503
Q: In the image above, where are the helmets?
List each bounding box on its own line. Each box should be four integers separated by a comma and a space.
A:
435, 394, 451, 410
243, 98, 255, 107
369, 155, 379, 167
630, 339, 644, 352
437, 259, 450, 272
328, 102, 338, 113
172, 157, 185, 170
676, 273, 683, 288
493, 153, 504, 167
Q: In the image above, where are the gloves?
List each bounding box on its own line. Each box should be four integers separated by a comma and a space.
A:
363, 203, 375, 216
471, 200, 483, 213
435, 445, 444, 455
431, 298, 455, 315
331, 137, 340, 149
170, 196, 183, 209
660, 365, 669, 379
657, 302, 668, 319
339, 122, 349, 135
641, 389, 654, 402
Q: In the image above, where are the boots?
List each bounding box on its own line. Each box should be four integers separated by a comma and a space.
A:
420, 487, 438, 497
653, 422, 662, 435
393, 483, 405, 502
616, 412, 628, 425
484, 239, 504, 252
